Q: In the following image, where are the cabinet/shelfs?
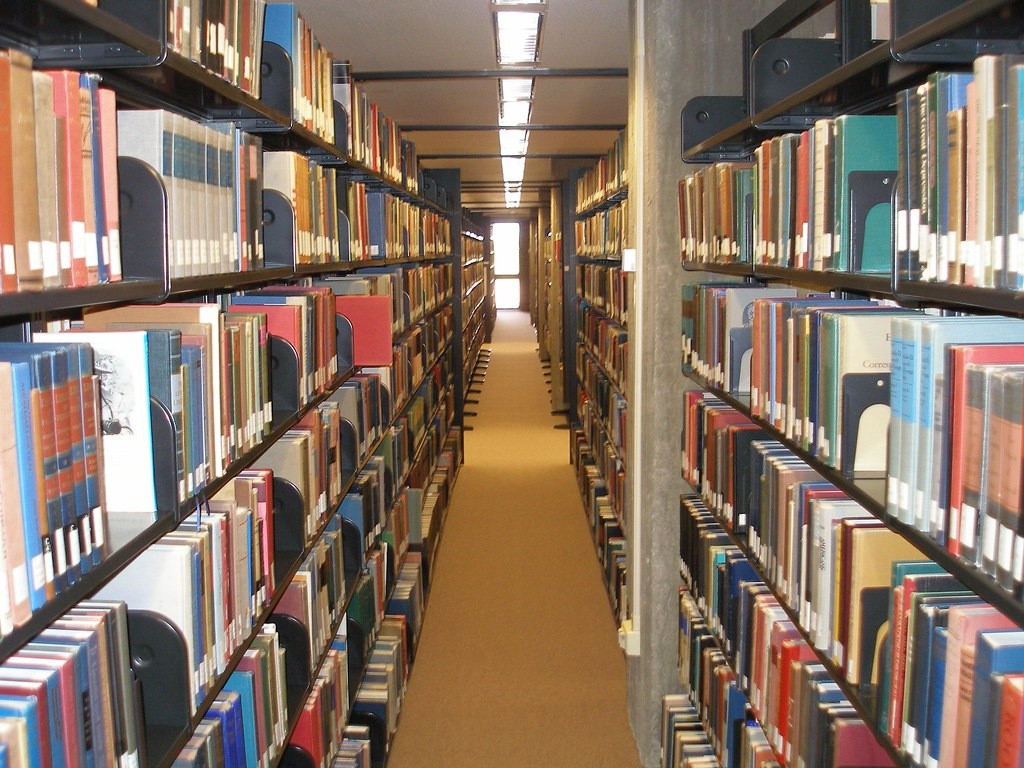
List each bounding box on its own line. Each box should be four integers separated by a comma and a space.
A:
527, 0, 1023, 768
0, 0, 494, 768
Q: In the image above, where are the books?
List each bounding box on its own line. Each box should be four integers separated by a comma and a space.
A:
0, 1, 497, 768
529, 1, 1024, 767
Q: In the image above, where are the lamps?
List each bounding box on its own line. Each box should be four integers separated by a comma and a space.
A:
487, 0, 549, 142
